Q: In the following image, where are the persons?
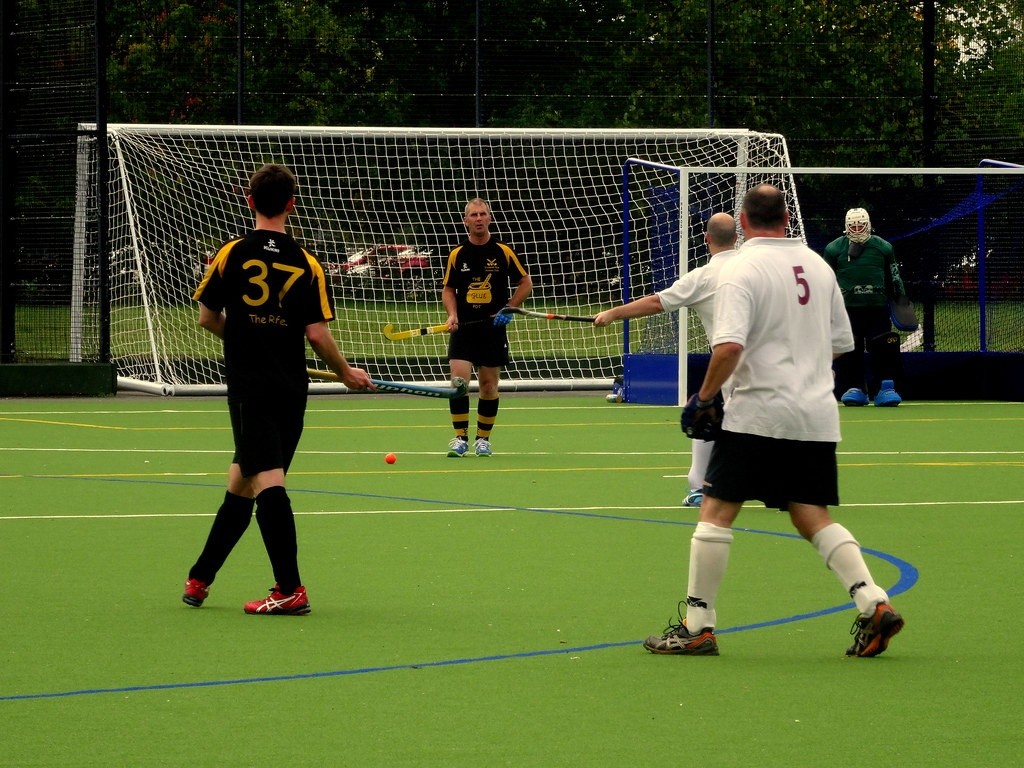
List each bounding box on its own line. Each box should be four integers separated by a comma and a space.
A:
441, 197, 533, 457
182, 163, 373, 614
823, 207, 919, 406
591, 212, 741, 508
645, 184, 904, 657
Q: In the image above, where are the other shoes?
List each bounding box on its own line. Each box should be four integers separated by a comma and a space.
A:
874, 380, 901, 407
841, 388, 869, 407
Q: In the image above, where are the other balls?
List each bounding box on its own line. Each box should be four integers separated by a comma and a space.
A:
386, 454, 396, 464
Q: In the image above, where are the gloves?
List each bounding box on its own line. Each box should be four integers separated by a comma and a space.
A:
489, 304, 514, 326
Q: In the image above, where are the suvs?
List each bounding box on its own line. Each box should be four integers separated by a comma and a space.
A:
329, 243, 434, 296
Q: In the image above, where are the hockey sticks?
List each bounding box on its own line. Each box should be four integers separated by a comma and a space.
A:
305, 366, 469, 399
501, 306, 596, 322
383, 317, 495, 340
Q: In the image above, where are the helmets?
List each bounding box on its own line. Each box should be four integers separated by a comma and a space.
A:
843, 207, 871, 245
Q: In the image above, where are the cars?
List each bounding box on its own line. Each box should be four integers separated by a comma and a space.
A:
942, 249, 1021, 300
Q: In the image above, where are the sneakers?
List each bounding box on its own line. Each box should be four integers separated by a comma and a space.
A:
681, 488, 704, 507
642, 602, 719, 656
446, 437, 469, 458
845, 603, 904, 658
242, 583, 311, 616
182, 577, 209, 607
472, 438, 492, 457
